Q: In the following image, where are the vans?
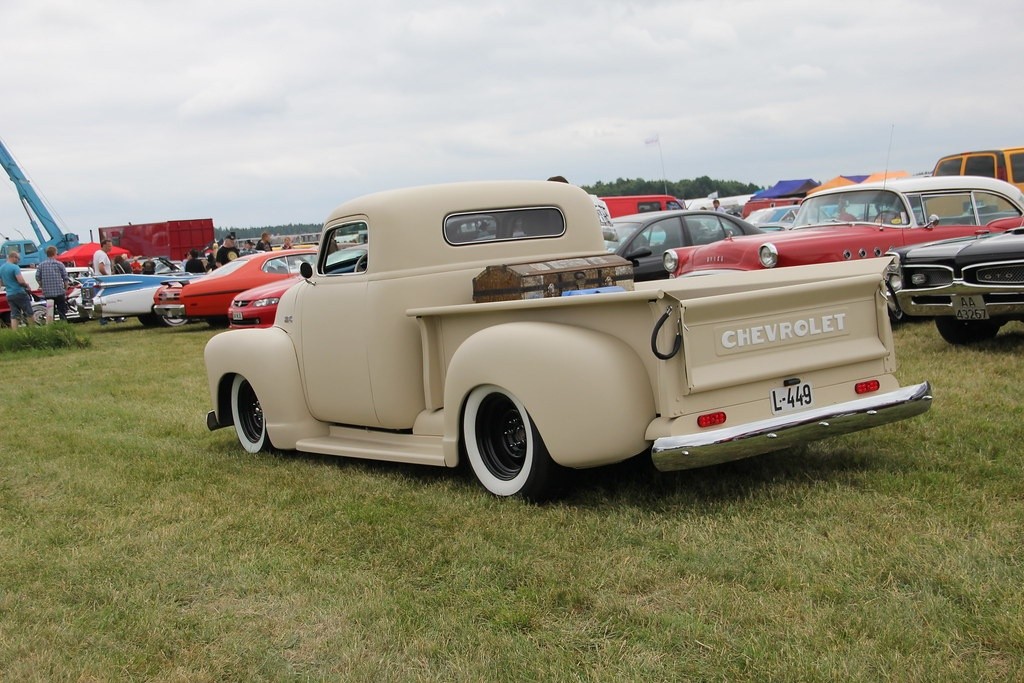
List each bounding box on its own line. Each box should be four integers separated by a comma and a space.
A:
600, 194, 686, 221
929, 147, 1024, 193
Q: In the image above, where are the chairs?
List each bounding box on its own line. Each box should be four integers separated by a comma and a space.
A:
633, 233, 651, 251
267, 266, 288, 274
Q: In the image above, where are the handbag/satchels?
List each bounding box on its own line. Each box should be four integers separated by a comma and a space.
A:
64, 303, 69, 313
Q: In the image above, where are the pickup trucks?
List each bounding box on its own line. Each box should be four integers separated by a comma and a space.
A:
203, 181, 935, 497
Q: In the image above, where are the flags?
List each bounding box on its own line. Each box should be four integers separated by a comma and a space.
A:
708, 191, 718, 200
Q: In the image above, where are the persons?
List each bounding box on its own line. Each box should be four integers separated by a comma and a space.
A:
712, 200, 726, 213
114, 255, 133, 274
282, 237, 295, 250
180, 234, 239, 274
93, 240, 123, 324
256, 233, 273, 252
242, 240, 255, 254
35, 246, 68, 323
0, 252, 34, 329
142, 255, 156, 275
131, 256, 143, 274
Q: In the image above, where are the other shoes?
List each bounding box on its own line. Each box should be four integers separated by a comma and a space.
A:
118, 319, 127, 321
100, 319, 106, 323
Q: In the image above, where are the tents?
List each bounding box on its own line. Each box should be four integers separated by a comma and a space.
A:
55, 242, 131, 267
749, 179, 819, 198
807, 172, 909, 196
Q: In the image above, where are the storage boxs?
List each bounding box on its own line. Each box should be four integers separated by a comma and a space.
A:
473, 251, 634, 303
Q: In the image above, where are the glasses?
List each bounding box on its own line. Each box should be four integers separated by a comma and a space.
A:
15, 257, 20, 260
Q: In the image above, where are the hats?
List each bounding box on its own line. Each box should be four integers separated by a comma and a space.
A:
245, 240, 255, 245
226, 234, 235, 239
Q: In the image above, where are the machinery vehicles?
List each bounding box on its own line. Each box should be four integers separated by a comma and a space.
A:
0, 140, 80, 267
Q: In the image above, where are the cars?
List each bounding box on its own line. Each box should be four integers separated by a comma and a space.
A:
0, 244, 317, 329
612, 176, 1024, 345
